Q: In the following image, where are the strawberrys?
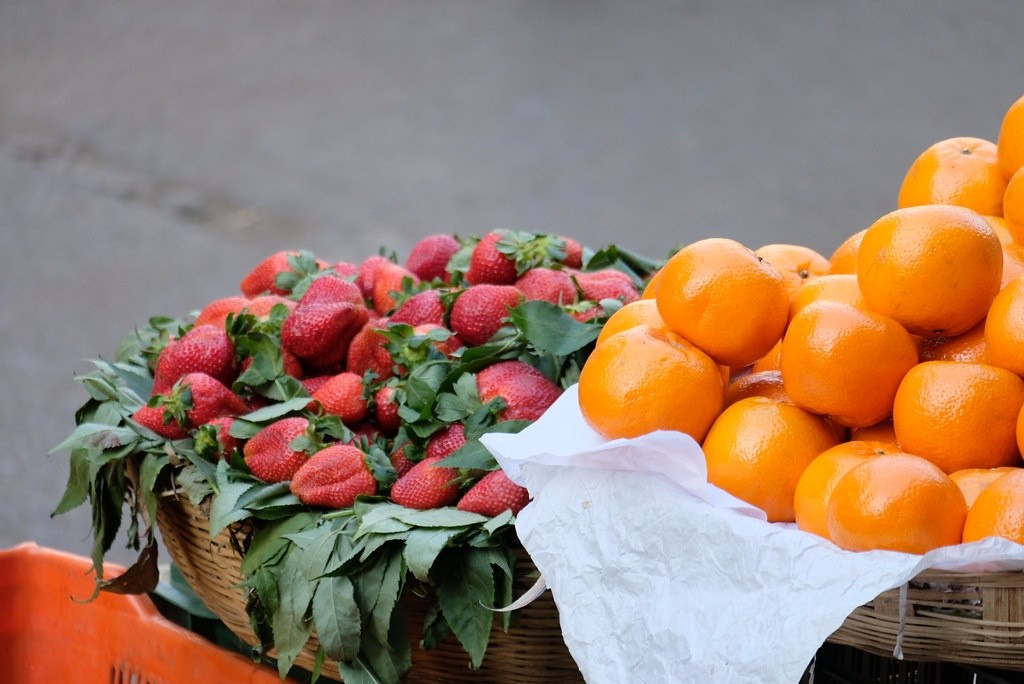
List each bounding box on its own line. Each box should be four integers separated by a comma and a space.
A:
128, 226, 640, 517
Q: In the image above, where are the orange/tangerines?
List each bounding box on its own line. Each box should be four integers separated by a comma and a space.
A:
578, 95, 1024, 554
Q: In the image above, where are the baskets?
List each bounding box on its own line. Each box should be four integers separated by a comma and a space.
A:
127, 449, 583, 684
827, 571, 1022, 666
